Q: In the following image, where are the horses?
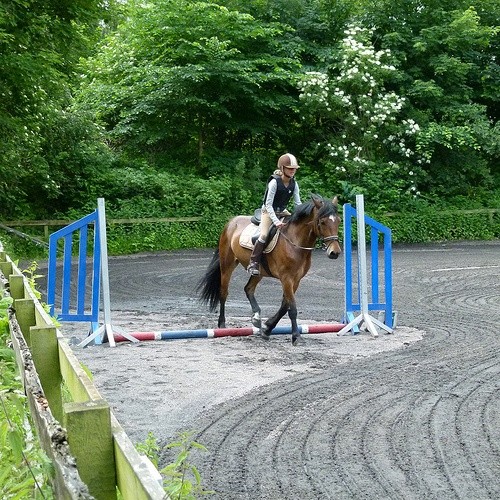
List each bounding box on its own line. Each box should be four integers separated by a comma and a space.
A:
190, 196, 342, 346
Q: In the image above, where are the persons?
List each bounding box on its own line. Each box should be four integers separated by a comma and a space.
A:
245, 153, 302, 277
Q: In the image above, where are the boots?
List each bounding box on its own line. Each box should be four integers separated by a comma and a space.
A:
247, 237, 266, 275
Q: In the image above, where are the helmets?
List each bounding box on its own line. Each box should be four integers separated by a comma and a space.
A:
278, 153, 300, 172
